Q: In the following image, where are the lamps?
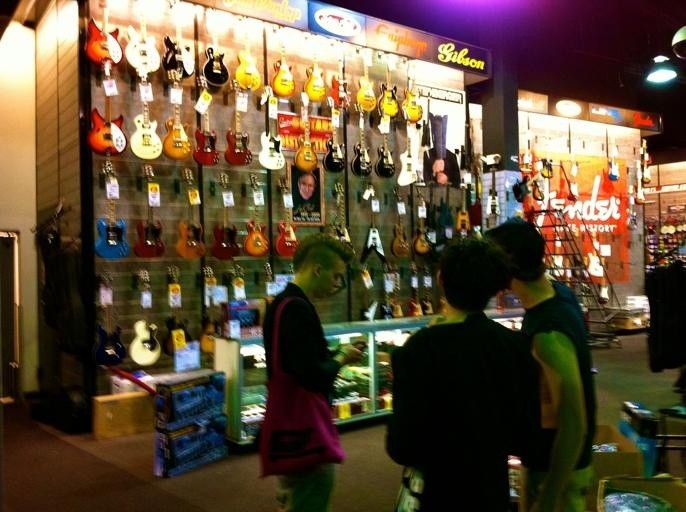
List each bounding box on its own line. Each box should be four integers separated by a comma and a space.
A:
646, 55, 678, 84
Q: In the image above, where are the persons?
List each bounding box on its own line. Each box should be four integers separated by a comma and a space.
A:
423, 115, 461, 188
294, 171, 318, 218
261, 230, 368, 511
483, 216, 598, 511
384, 238, 543, 511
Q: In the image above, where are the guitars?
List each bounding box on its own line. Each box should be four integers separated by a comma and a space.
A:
587, 227, 604, 279
513, 171, 541, 202
94, 269, 128, 367
201, 262, 297, 354
225, 79, 254, 166
300, 91, 318, 172
191, 74, 220, 166
174, 167, 207, 261
597, 255, 610, 304
258, 85, 286, 170
162, 71, 195, 160
608, 137, 619, 180
275, 175, 299, 257
211, 172, 242, 260
375, 114, 396, 177
129, 269, 163, 366
87, 58, 128, 156
242, 172, 272, 258
328, 177, 471, 322
270, 25, 423, 123
163, 265, 195, 355
83, 1, 262, 92
396, 106, 418, 186
133, 163, 166, 259
93, 161, 130, 259
633, 160, 646, 206
130, 69, 163, 161
321, 96, 346, 173
350, 103, 372, 176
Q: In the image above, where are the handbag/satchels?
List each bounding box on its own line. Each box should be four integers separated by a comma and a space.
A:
257, 377, 348, 479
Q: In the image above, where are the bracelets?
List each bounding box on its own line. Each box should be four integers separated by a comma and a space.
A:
338, 350, 351, 365
